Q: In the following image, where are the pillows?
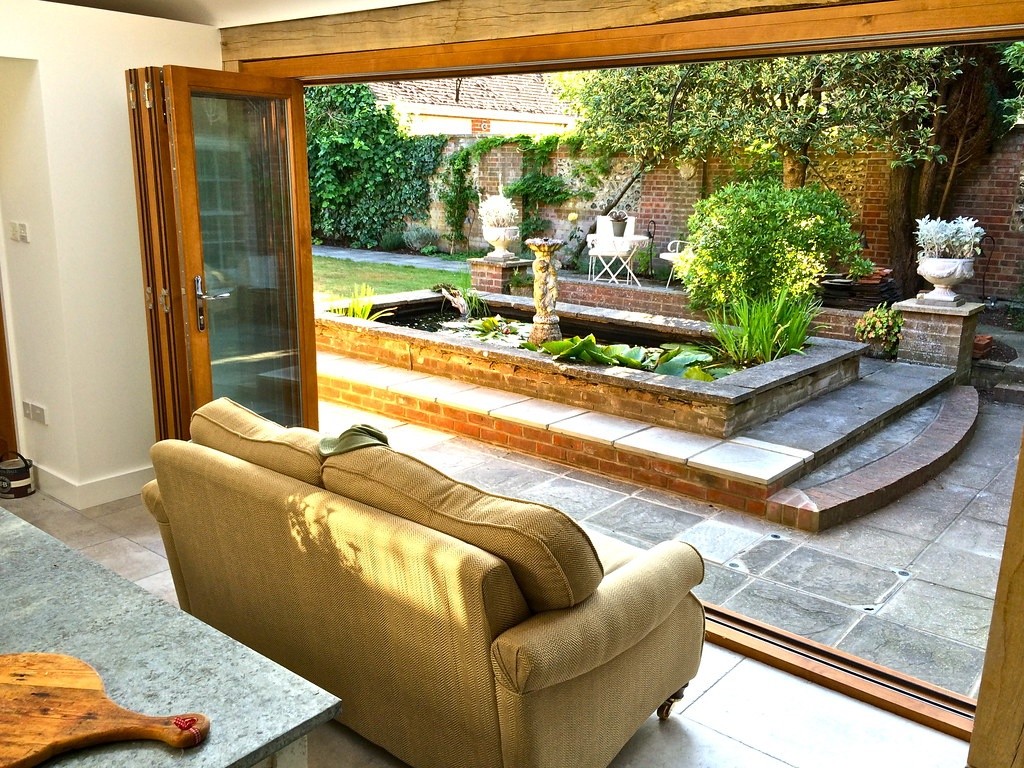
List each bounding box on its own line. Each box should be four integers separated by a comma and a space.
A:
188, 396, 328, 489
321, 444, 605, 613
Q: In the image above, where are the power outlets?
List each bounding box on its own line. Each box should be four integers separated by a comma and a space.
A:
31, 404, 45, 424
23, 401, 32, 419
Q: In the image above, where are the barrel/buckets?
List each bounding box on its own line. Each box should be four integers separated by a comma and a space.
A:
0, 450, 37, 499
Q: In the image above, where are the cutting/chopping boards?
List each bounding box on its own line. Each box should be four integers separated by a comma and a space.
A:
0, 652, 212, 768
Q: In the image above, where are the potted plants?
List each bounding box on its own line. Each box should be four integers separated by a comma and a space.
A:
608, 211, 628, 236
911, 214, 986, 302
854, 301, 905, 360
508, 265, 534, 297
479, 195, 520, 257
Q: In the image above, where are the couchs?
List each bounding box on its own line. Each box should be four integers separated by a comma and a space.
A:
139, 398, 705, 768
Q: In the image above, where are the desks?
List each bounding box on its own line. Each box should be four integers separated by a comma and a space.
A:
587, 234, 649, 287
0, 506, 342, 768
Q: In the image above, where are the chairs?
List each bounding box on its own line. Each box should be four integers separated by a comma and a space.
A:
659, 240, 691, 288
588, 215, 635, 285
